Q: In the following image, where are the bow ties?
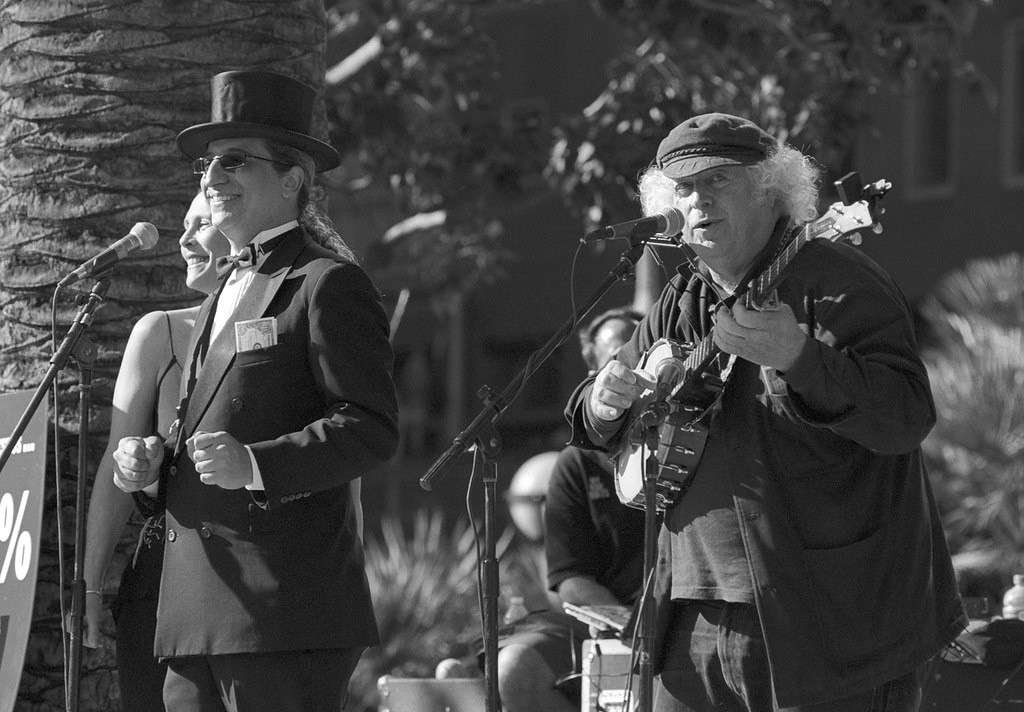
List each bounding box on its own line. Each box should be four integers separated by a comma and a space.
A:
214, 242, 255, 281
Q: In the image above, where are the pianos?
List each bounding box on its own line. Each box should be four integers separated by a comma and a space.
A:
562, 598, 638, 711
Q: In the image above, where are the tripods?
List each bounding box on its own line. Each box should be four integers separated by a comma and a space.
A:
412, 242, 651, 712
0, 278, 113, 712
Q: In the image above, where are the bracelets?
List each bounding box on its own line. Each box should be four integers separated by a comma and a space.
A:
85, 590, 103, 602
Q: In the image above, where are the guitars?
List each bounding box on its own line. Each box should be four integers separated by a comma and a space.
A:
607, 171, 892, 516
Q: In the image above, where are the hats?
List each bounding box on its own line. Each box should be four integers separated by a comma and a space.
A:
655, 112, 778, 179
174, 70, 342, 174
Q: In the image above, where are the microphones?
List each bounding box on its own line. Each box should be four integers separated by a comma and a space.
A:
580, 207, 686, 246
56, 221, 161, 290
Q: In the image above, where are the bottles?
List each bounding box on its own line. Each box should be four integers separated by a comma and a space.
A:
504, 597, 529, 625
1001, 574, 1023, 623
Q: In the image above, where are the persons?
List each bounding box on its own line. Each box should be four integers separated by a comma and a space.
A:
496, 306, 646, 712
974, 617, 1024, 710
563, 113, 972, 712
112, 70, 401, 711
62, 185, 360, 711
